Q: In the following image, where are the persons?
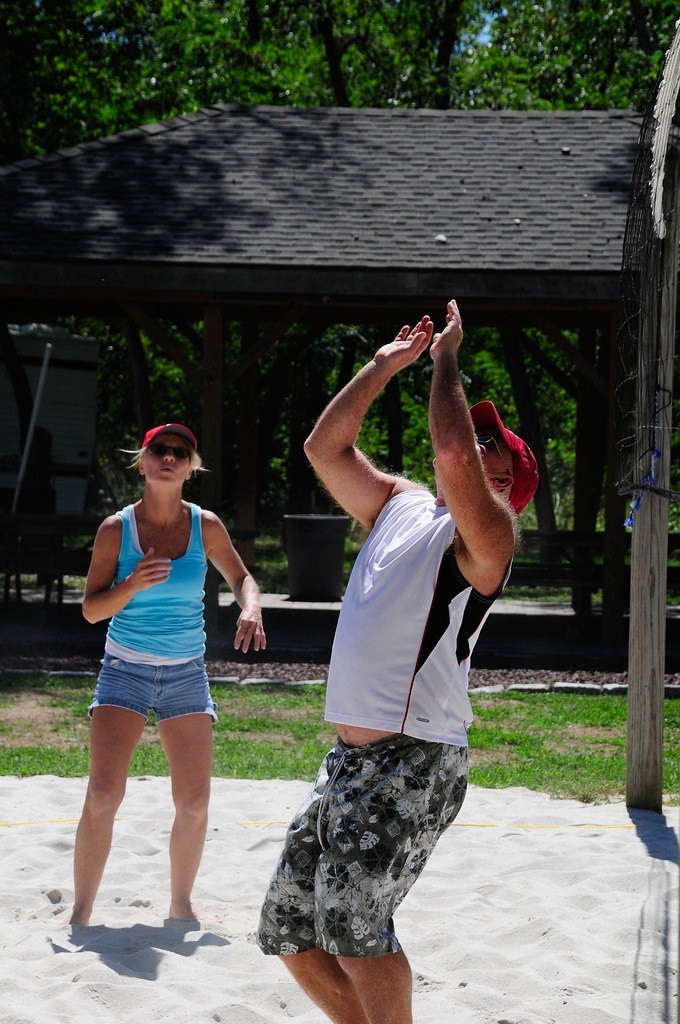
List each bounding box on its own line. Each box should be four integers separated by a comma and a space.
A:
70, 424, 266, 927
256, 299, 540, 1024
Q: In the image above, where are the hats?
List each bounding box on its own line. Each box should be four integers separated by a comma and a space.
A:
142, 424, 197, 452
468, 401, 539, 514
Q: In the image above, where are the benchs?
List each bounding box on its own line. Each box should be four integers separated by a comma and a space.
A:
505, 528, 680, 639
0, 512, 113, 616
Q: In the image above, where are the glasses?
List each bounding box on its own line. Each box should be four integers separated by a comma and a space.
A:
148, 444, 190, 459
477, 432, 512, 477
